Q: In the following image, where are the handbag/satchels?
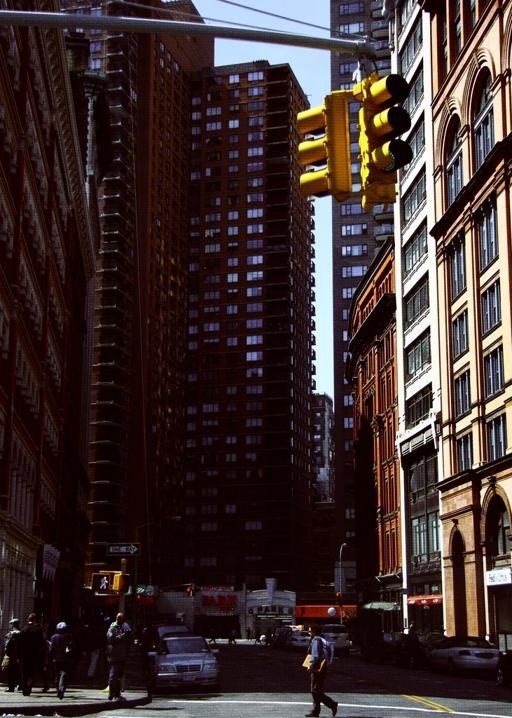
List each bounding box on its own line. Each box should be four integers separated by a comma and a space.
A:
302, 654, 326, 672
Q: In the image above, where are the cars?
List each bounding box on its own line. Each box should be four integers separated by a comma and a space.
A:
151, 634, 217, 692
430, 636, 500, 673
290, 623, 352, 649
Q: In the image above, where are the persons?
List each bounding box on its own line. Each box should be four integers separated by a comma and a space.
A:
208, 625, 266, 646
2, 612, 135, 701
304, 623, 339, 718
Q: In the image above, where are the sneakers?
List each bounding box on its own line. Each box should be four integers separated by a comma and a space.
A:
332, 701, 339, 717
304, 713, 319, 718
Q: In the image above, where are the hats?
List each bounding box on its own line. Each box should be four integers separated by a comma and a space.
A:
56, 621, 68, 631
9, 618, 20, 624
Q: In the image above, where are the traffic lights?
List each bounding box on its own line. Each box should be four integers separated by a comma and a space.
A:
295, 89, 351, 205
183, 583, 193, 598
351, 72, 413, 210
89, 570, 129, 598
